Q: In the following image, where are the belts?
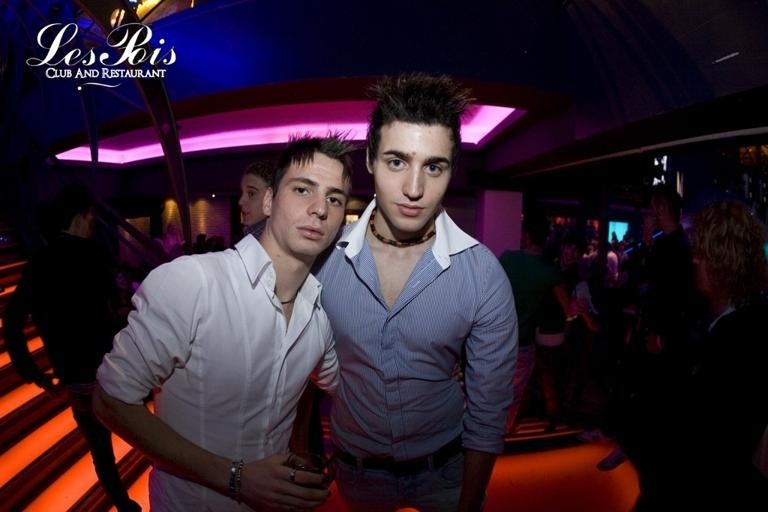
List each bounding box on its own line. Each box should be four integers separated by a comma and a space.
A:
329, 432, 461, 473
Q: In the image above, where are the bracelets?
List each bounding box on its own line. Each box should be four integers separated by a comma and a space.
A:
228, 456, 243, 504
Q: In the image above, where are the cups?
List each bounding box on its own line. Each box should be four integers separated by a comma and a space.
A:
286, 451, 339, 491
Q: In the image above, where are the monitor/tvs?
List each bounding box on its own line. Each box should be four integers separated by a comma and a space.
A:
608, 221, 629, 244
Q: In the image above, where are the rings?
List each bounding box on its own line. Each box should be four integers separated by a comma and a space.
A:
290, 469, 298, 482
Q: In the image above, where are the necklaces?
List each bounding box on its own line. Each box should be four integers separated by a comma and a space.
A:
368, 207, 436, 248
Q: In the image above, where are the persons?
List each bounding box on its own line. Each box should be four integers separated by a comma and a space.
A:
499, 181, 767, 512
90, 128, 340, 512
164, 221, 185, 256
2, 186, 143, 512
238, 159, 273, 241
311, 75, 519, 512
